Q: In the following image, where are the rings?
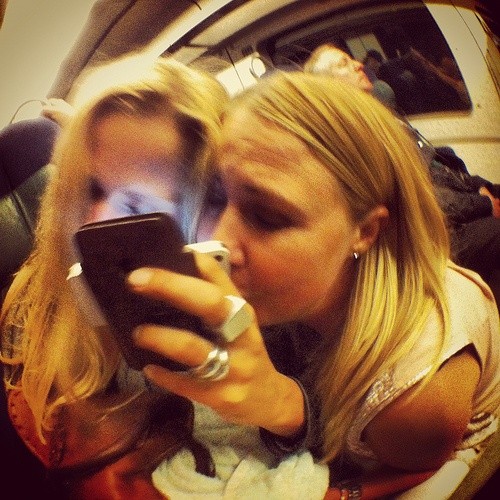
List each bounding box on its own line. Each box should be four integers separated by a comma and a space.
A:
210, 296, 258, 346
188, 344, 230, 381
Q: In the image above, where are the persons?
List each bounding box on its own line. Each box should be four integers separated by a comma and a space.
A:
0, 59, 500, 500
354, 16, 471, 116
304, 43, 500, 310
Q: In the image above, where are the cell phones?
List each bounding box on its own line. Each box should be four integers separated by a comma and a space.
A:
187, 241, 231, 277
75, 211, 212, 373
